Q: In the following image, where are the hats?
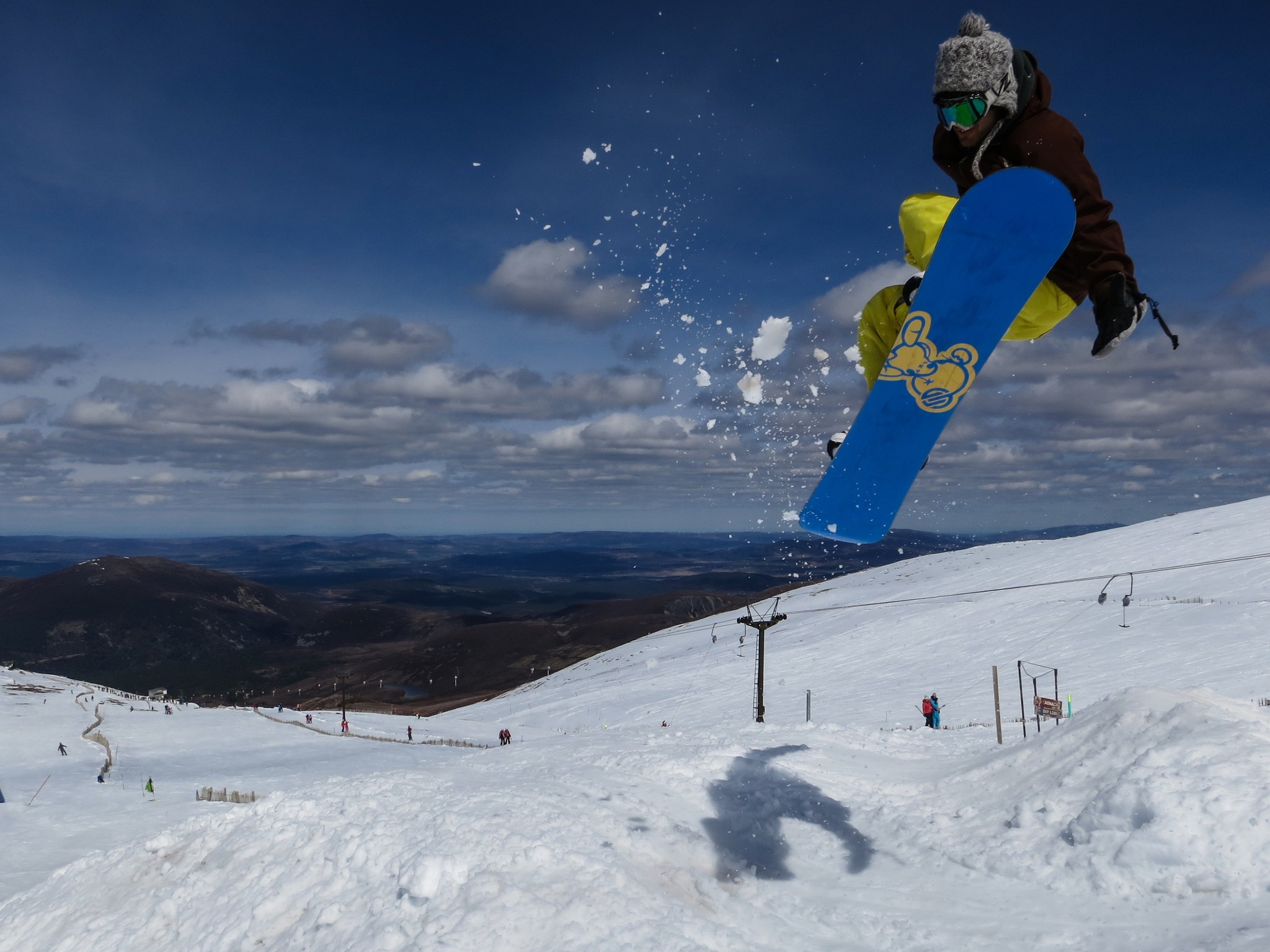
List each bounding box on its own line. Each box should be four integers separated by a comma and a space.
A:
924, 695, 930, 699
932, 693, 937, 696
934, 10, 1019, 118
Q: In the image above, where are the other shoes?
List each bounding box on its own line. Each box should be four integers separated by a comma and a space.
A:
826, 430, 929, 468
1091, 272, 1149, 360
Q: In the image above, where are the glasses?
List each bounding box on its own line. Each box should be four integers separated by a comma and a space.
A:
936, 63, 1016, 131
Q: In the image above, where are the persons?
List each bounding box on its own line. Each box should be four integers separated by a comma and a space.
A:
308, 714, 312, 723
58, 742, 67, 756
823, 9, 1147, 467
407, 725, 413, 741
253, 702, 301, 712
503, 728, 511, 744
341, 719, 345, 732
344, 721, 349, 732
42, 689, 94, 704
499, 729, 506, 745
306, 714, 310, 724
95, 685, 188, 715
923, 695, 935, 728
929, 693, 941, 729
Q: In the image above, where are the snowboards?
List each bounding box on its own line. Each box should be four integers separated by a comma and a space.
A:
798, 165, 1079, 548
